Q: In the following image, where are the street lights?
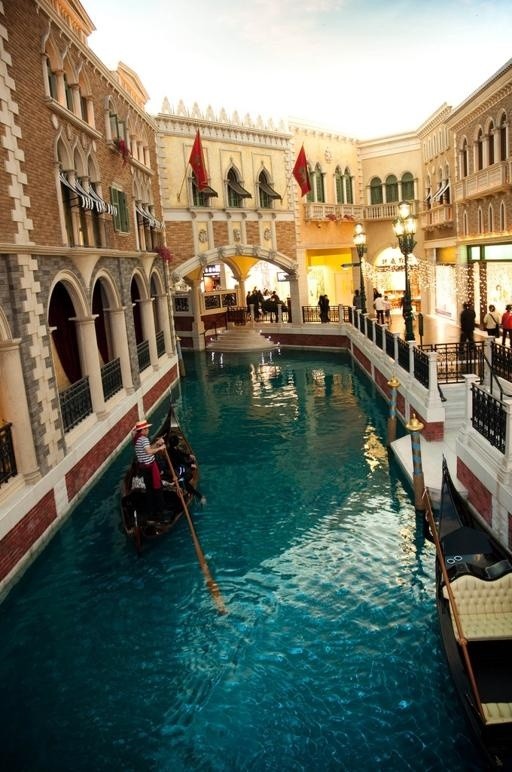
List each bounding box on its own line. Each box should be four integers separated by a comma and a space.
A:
352, 219, 367, 314
391, 201, 419, 340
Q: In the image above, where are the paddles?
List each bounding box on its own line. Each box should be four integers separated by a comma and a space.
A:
421, 487, 485, 721
158, 434, 230, 617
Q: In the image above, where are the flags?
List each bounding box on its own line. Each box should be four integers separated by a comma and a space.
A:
188, 129, 210, 194
291, 146, 313, 198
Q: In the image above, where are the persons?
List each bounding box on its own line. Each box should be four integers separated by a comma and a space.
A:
131, 420, 169, 491
383, 296, 392, 324
501, 305, 511, 349
482, 303, 501, 338
318, 294, 329, 306
372, 293, 385, 324
399, 290, 406, 325
456, 301, 476, 354
246, 286, 284, 324
160, 434, 206, 506
373, 288, 379, 301
352, 290, 361, 309
317, 296, 328, 322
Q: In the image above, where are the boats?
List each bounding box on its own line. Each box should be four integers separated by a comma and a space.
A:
113, 403, 203, 560
428, 450, 511, 772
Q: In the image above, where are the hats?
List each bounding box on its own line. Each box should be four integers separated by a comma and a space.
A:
133, 419, 153, 431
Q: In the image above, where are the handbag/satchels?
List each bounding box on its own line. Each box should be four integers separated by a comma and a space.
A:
495, 323, 499, 337
